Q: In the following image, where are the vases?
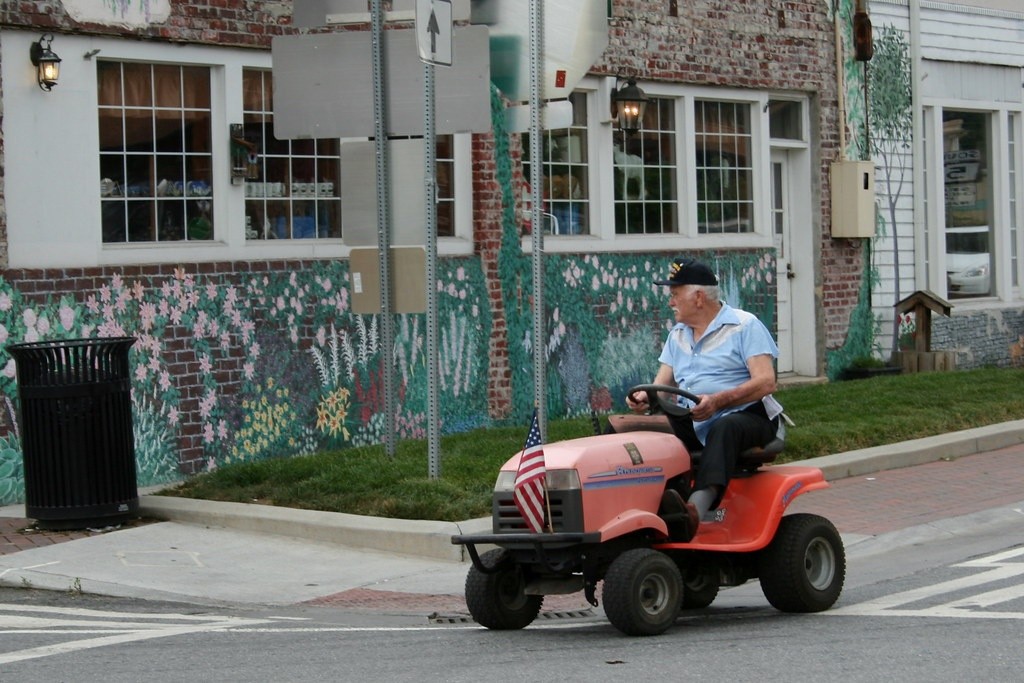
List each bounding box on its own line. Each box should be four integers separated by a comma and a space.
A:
891, 351, 903, 366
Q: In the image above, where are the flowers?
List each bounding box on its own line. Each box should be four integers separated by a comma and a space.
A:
896, 313, 916, 351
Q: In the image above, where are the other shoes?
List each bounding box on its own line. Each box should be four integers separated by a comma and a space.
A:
660, 489, 700, 543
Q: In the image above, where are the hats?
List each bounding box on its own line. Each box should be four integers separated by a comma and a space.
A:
653, 257, 719, 285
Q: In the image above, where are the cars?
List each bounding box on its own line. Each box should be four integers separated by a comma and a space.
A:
946, 230, 992, 297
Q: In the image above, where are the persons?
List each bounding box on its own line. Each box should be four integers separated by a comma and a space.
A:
602, 256, 786, 542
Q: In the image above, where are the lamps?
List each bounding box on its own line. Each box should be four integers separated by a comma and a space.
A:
30, 31, 62, 91
600, 74, 650, 134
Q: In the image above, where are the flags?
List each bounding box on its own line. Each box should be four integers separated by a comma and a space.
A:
512, 408, 548, 534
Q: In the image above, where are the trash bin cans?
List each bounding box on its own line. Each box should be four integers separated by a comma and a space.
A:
5, 336, 143, 525
